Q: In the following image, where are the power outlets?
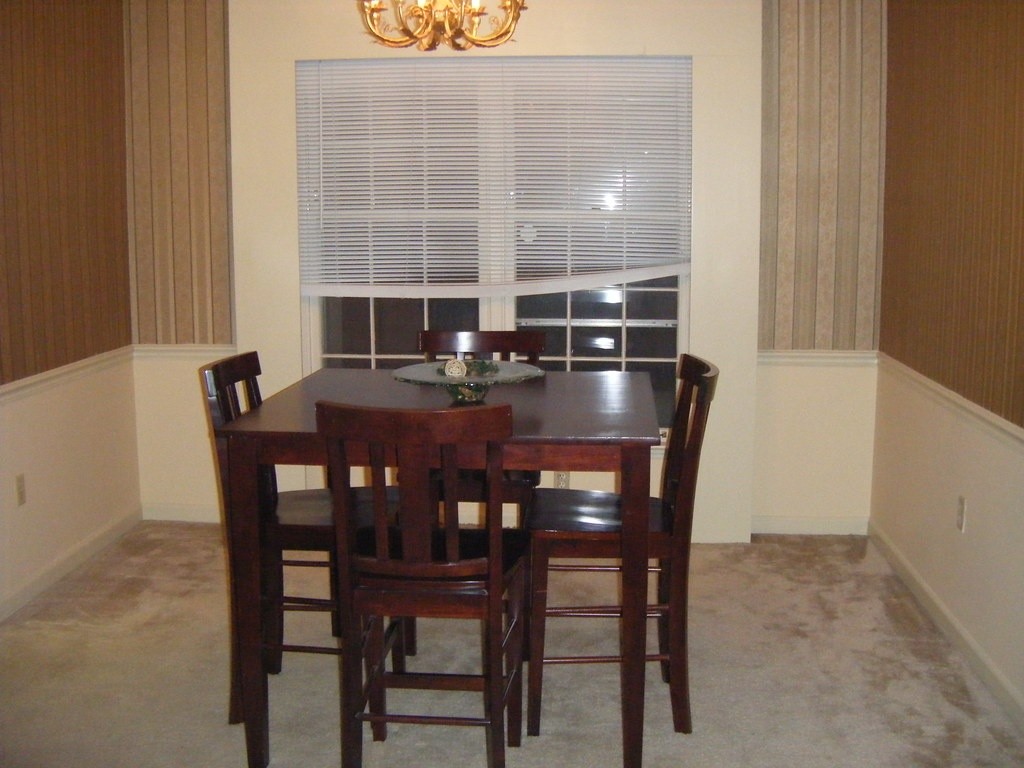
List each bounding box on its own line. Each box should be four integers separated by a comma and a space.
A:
17, 473, 26, 506
956, 495, 966, 533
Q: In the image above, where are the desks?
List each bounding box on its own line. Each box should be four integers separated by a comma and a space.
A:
215, 368, 661, 768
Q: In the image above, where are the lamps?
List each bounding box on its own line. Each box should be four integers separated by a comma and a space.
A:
356, 0, 528, 51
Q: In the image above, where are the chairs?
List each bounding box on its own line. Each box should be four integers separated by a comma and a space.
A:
314, 399, 528, 768
419, 331, 547, 658
521, 353, 719, 737
199, 350, 417, 726
